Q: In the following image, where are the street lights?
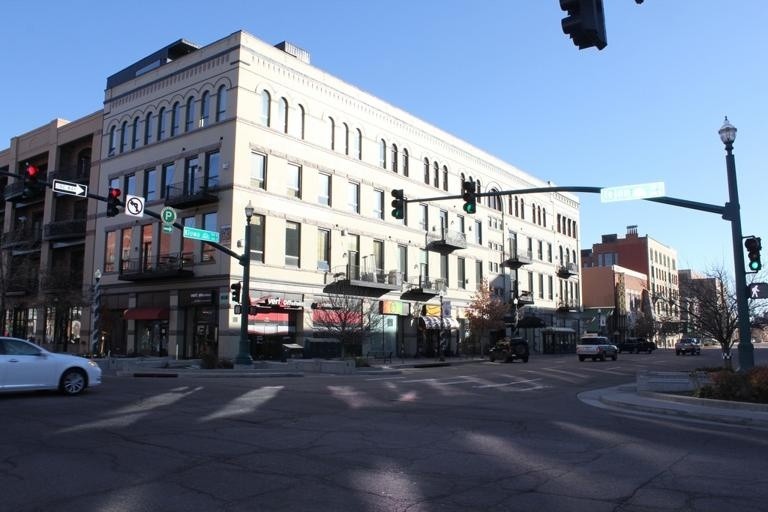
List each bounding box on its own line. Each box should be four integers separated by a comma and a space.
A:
90, 267, 104, 355
512, 297, 520, 335
596, 307, 602, 333
715, 113, 754, 371
238, 198, 257, 364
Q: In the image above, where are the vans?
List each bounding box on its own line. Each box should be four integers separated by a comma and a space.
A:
703, 338, 717, 344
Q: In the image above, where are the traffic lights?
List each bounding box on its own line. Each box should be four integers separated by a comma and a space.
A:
463, 181, 475, 213
746, 238, 759, 269
250, 306, 257, 314
24, 164, 36, 197
231, 283, 239, 301
109, 188, 120, 218
390, 189, 402, 219
750, 284, 767, 298
555, 0, 571, 37
234, 305, 241, 313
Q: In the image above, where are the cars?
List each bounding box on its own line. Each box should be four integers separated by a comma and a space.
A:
0, 335, 103, 398
141, 332, 159, 351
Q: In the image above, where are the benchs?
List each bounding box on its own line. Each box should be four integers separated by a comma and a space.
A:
366, 351, 394, 364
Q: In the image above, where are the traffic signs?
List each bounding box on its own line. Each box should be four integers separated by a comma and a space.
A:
601, 182, 666, 202
53, 179, 88, 197
184, 227, 221, 243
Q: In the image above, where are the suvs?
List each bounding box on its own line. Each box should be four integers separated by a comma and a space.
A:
576, 335, 619, 364
623, 338, 657, 355
488, 335, 531, 364
675, 339, 701, 355
